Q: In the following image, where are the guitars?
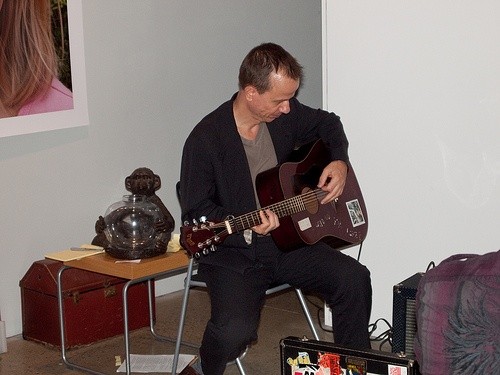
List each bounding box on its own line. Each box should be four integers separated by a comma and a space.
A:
180, 136, 368, 260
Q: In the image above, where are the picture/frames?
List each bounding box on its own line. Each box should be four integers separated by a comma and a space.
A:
0, 0, 90, 138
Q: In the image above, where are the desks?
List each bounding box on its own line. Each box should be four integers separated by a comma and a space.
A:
45, 247, 199, 375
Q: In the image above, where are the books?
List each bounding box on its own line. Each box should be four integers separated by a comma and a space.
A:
45, 245, 106, 263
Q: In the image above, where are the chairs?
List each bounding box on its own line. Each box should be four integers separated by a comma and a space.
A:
170, 258, 320, 375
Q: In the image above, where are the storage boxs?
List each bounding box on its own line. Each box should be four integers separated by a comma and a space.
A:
18, 260, 156, 353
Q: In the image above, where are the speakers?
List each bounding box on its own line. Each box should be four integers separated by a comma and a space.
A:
391, 272, 427, 356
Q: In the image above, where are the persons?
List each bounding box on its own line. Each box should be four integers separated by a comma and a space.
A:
0, 1, 74, 117
181, 42, 372, 375
